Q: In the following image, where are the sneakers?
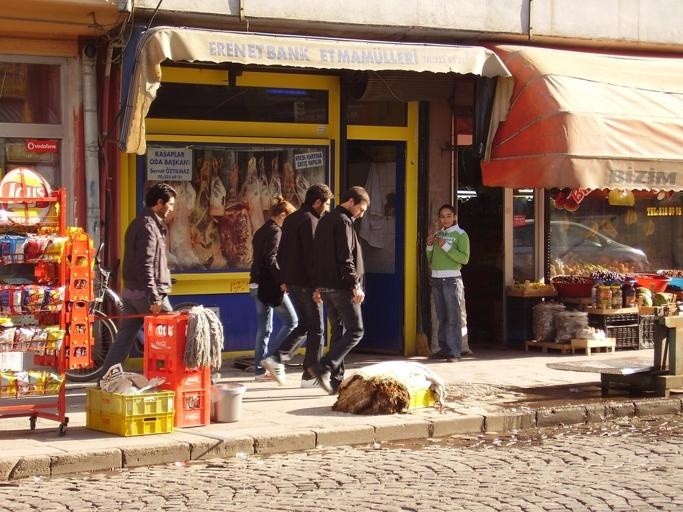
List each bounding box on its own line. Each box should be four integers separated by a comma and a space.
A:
301, 363, 332, 393
255, 357, 286, 386
429, 353, 458, 362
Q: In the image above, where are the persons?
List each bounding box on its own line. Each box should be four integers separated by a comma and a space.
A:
259, 182, 332, 388
94, 182, 177, 388
248, 195, 297, 382
426, 276, 474, 359
425, 205, 470, 364
307, 184, 371, 394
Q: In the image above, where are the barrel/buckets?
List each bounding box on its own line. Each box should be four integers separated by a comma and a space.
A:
211, 383, 245, 423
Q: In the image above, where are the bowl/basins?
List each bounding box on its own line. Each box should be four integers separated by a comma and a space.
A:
668, 276, 682, 290
636, 274, 670, 295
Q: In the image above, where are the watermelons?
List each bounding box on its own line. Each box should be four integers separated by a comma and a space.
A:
636, 287, 652, 307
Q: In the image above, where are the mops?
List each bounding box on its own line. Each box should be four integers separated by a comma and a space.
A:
93, 304, 224, 369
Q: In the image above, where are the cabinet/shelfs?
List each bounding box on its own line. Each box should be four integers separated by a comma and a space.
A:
507, 277, 654, 317
0, 187, 95, 435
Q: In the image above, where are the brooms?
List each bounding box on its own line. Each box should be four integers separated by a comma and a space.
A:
416, 241, 434, 357
